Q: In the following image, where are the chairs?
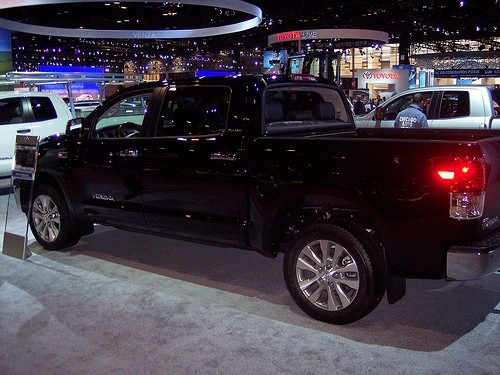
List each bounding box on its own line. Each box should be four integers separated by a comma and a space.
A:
164, 108, 197, 135
9, 104, 24, 124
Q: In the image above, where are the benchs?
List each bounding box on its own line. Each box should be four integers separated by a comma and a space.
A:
265, 101, 344, 127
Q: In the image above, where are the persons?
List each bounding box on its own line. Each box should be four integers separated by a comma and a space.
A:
345, 95, 385, 115
393, 93, 428, 127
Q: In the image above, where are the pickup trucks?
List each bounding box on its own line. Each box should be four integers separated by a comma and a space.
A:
353, 84, 500, 130
0, 90, 146, 191
12, 69, 499, 327
